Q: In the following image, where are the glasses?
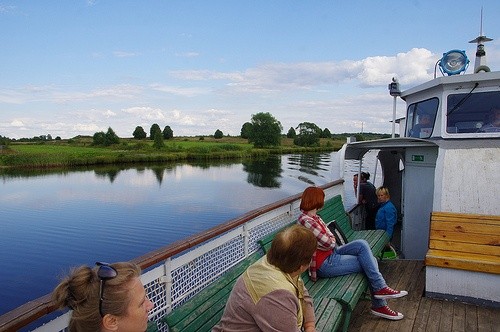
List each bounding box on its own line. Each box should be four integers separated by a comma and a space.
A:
95, 261, 118, 317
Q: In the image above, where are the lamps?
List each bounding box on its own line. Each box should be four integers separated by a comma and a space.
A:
439, 50, 471, 77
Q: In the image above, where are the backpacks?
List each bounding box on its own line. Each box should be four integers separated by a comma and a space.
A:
324, 219, 349, 248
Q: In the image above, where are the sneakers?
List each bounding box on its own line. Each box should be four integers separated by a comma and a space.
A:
370, 305, 404, 320
374, 287, 408, 299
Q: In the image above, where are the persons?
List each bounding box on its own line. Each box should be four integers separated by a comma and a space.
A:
483, 102, 500, 128
51, 261, 153, 332
411, 113, 433, 137
212, 224, 318, 332
295, 187, 408, 320
353, 172, 378, 230
376, 186, 397, 252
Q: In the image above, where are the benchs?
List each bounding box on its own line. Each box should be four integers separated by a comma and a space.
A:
162, 251, 350, 332
424, 212, 500, 275
258, 194, 391, 312
